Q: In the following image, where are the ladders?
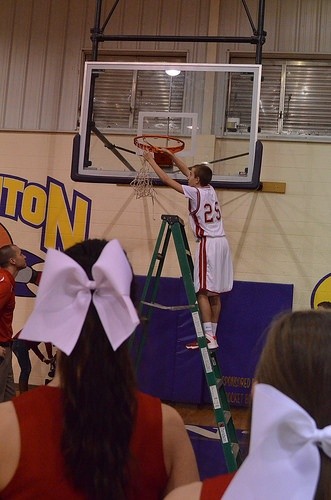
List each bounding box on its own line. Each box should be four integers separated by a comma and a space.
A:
129, 214, 243, 473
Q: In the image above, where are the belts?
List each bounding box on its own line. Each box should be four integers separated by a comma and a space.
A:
0, 341, 12, 347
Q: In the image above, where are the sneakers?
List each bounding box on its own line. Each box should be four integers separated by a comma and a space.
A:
185, 336, 219, 350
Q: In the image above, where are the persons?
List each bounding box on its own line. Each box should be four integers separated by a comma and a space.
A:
0, 238, 200, 500
165, 302, 331, 500
142, 146, 233, 349
